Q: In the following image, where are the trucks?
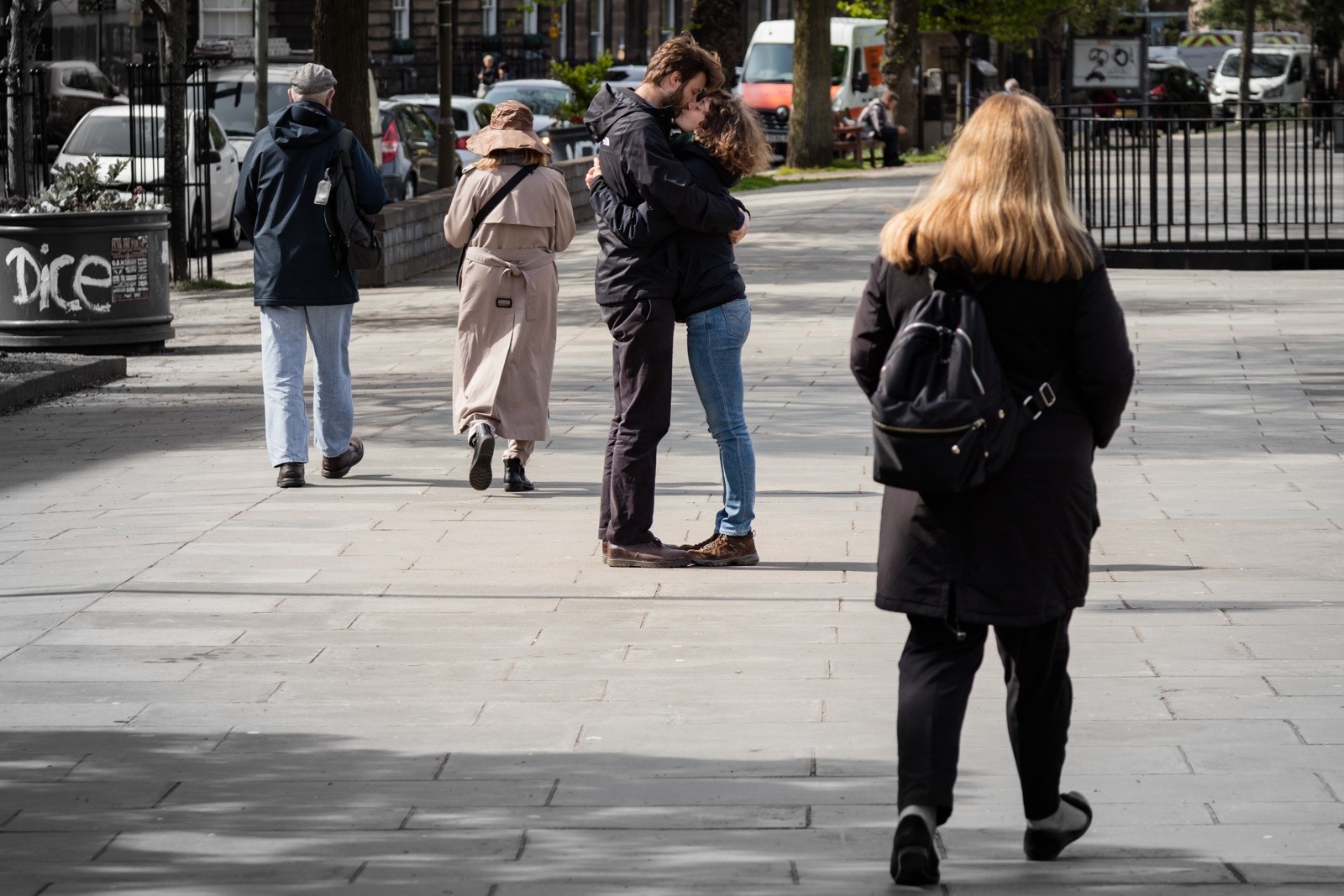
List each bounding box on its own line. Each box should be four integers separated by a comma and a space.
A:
1209, 47, 1321, 123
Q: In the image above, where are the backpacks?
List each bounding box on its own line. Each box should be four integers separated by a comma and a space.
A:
871, 255, 1090, 495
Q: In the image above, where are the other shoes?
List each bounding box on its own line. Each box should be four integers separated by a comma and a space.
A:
889, 814, 942, 890
1315, 136, 1321, 148
1023, 790, 1093, 861
882, 159, 904, 167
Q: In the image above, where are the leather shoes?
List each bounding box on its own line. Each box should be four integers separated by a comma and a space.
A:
503, 457, 534, 492
602, 535, 690, 568
276, 463, 305, 488
322, 436, 365, 478
468, 423, 495, 490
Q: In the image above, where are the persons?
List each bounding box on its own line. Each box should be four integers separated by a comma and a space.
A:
476, 55, 512, 99
1086, 81, 1171, 148
444, 99, 576, 492
849, 93, 1132, 886
1005, 78, 1021, 92
858, 91, 908, 167
584, 31, 772, 568
234, 63, 388, 488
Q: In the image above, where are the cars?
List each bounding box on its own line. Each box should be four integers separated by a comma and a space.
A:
1092, 62, 1211, 133
33, 58, 135, 143
482, 78, 578, 147
388, 94, 496, 170
598, 63, 650, 92
49, 106, 250, 251
377, 99, 467, 201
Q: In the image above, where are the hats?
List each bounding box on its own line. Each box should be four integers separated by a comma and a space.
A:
467, 99, 554, 157
291, 63, 338, 95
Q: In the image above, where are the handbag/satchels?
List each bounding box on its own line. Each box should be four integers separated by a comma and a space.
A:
476, 84, 489, 98
313, 127, 382, 273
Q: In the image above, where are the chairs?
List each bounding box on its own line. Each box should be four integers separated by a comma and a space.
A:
102, 123, 129, 153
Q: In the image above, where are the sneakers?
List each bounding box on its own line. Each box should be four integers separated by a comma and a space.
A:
680, 531, 759, 566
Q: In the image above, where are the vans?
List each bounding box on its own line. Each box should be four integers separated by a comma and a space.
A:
733, 16, 889, 158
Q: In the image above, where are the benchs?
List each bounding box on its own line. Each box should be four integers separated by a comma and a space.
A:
832, 112, 885, 169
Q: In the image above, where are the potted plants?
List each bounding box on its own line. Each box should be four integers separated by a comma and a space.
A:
0, 150, 176, 354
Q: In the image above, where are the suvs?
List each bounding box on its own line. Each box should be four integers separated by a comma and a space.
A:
184, 48, 384, 176
